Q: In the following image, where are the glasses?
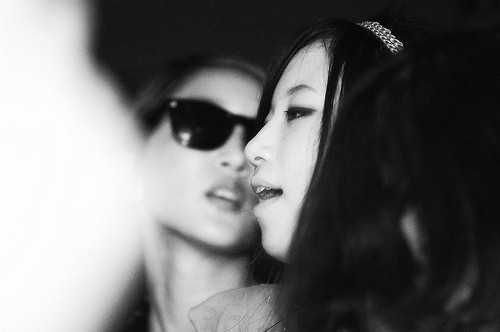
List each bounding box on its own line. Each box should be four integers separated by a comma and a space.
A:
149, 97, 259, 151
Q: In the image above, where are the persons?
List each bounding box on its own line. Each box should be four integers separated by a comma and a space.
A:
190, 17, 497, 331
112, 45, 276, 332
